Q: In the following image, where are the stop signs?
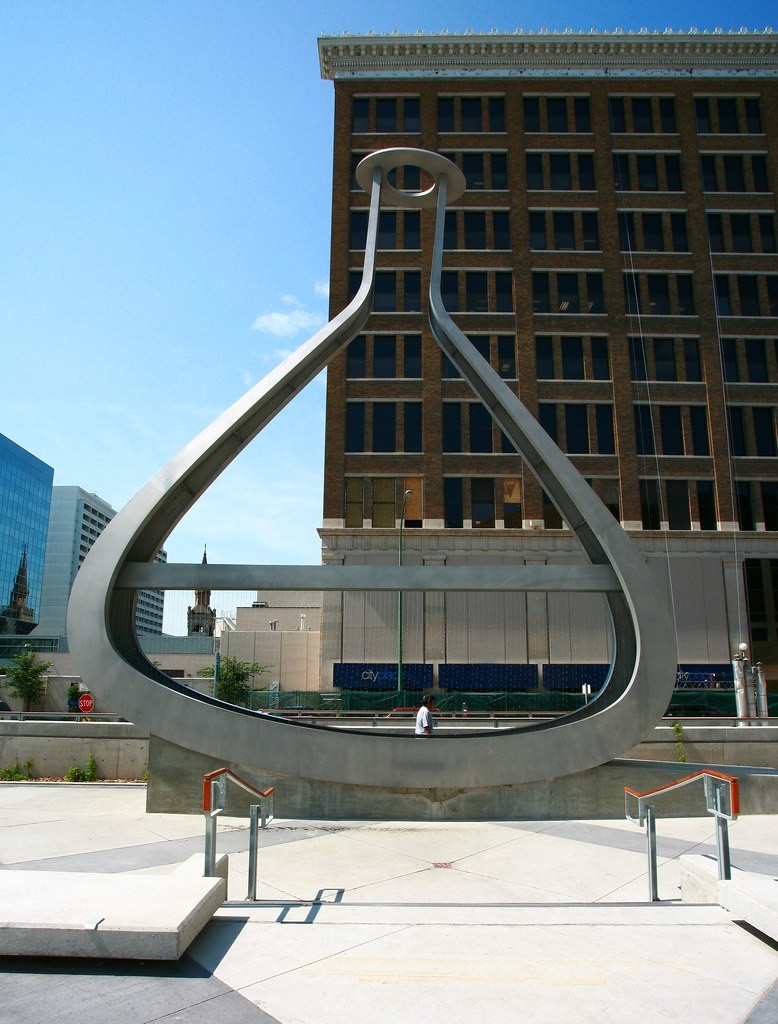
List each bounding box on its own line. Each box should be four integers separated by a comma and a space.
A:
79, 694, 93, 713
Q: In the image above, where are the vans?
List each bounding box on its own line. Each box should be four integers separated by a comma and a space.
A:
385, 708, 442, 718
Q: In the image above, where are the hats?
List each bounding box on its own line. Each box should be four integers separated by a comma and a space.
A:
420, 695, 435, 703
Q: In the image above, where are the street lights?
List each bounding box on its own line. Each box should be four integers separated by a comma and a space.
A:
396, 490, 412, 687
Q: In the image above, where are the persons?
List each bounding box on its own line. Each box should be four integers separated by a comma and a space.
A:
415, 694, 435, 735
